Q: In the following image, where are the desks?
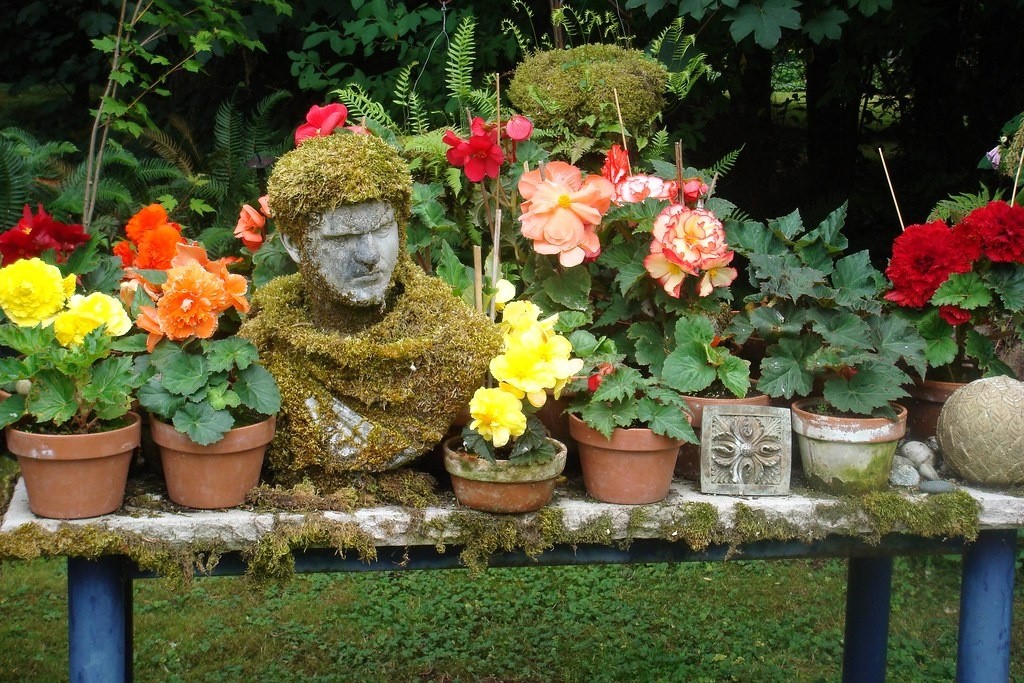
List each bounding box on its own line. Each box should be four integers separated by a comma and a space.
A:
1, 474, 1022, 683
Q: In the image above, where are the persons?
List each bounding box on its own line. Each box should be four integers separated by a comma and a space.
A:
233, 135, 510, 474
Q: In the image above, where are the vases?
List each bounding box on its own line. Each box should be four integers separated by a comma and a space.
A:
569, 412, 693, 506
747, 247, 930, 497
149, 414, 277, 511
5, 408, 142, 521
678, 380, 770, 484
906, 366, 990, 442
536, 373, 593, 457
443, 438, 568, 514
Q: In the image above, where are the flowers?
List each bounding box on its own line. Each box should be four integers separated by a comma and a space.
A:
0, 90, 756, 466
883, 199, 1024, 381
